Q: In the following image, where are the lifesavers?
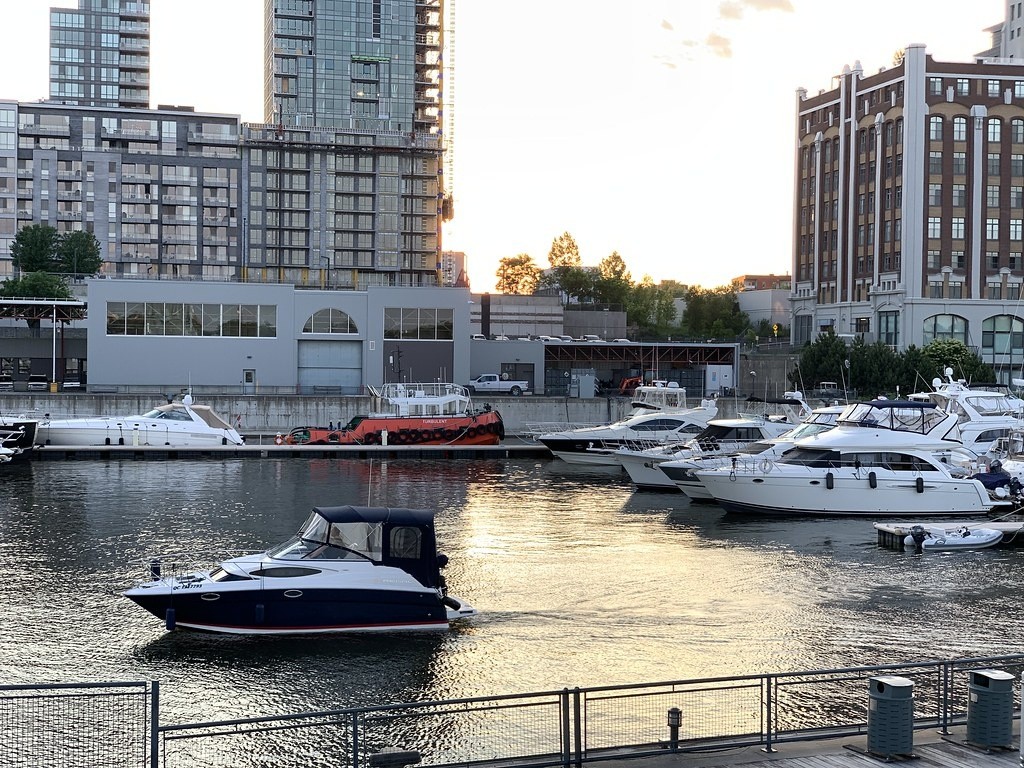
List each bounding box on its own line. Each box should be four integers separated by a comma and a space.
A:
697, 437, 707, 453
486, 420, 505, 442
408, 429, 433, 442
758, 459, 774, 474
710, 435, 720, 450
467, 424, 486, 439
703, 436, 713, 452
273, 436, 284, 445
432, 426, 466, 442
363, 429, 407, 444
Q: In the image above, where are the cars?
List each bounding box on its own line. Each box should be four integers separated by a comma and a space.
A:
583, 335, 606, 343
470, 334, 487, 340
517, 338, 531, 341
535, 335, 575, 342
612, 338, 630, 342
493, 336, 510, 341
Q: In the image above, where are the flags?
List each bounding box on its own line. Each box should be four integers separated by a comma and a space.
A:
237, 414, 241, 429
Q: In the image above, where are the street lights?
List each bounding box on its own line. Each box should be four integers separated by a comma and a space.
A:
603, 308, 610, 341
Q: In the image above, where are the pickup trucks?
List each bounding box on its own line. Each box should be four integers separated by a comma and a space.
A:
470, 373, 529, 396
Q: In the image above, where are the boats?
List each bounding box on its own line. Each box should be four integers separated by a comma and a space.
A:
904, 523, 1004, 551
33, 385, 246, 446
523, 366, 1024, 517
120, 504, 477, 635
0, 409, 40, 450
282, 366, 506, 447
